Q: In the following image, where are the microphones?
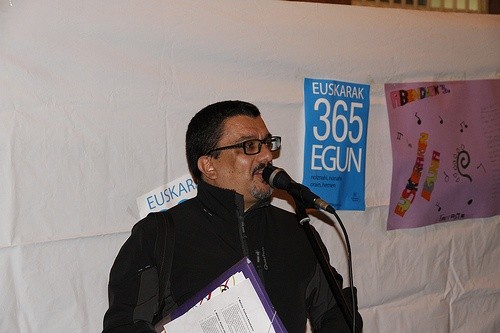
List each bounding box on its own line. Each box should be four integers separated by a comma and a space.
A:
262, 165, 335, 214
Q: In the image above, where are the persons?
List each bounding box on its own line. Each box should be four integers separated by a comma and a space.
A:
101, 100, 363, 333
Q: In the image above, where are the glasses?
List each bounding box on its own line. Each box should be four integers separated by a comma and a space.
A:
206, 136, 281, 155
